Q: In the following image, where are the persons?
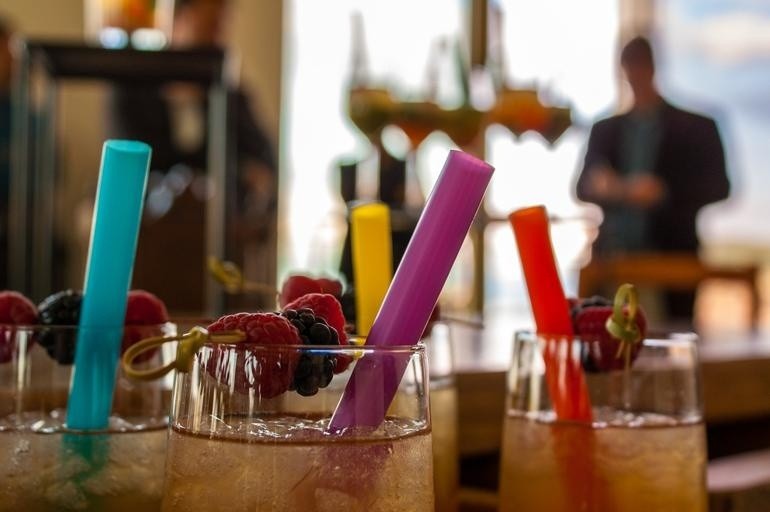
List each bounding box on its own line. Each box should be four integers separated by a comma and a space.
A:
114, 2, 283, 310
569, 33, 732, 336
331, 135, 420, 282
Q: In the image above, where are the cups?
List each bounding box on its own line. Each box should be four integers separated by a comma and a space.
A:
497, 330, 709, 510
5, 323, 176, 512
166, 321, 459, 512
